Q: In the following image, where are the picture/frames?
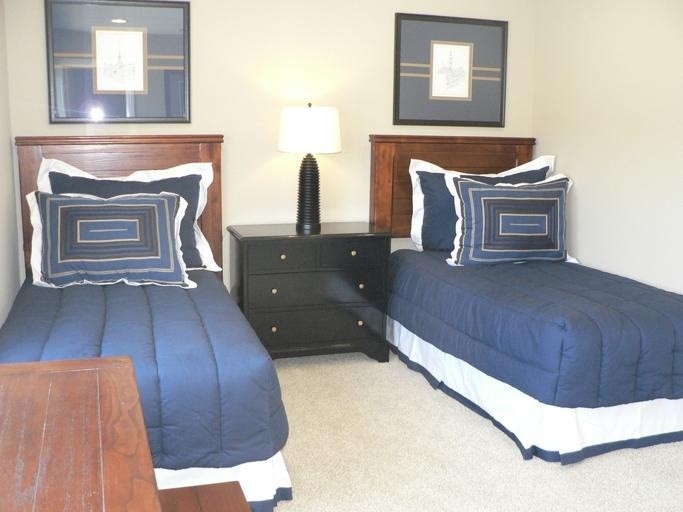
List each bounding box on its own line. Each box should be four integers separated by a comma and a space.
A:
392, 12, 508, 128
42, 0, 193, 126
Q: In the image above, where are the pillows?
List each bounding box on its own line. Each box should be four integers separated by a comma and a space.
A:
20, 152, 224, 289
401, 152, 572, 263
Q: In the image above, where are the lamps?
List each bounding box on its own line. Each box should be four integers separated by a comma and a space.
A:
277, 105, 343, 234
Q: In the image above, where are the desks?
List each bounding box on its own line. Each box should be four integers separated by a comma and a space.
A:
0, 352, 162, 512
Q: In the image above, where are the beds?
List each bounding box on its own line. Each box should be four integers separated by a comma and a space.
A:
368, 130, 682, 459
2, 134, 300, 512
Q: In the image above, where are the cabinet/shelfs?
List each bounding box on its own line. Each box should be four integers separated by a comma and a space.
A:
228, 223, 398, 365
